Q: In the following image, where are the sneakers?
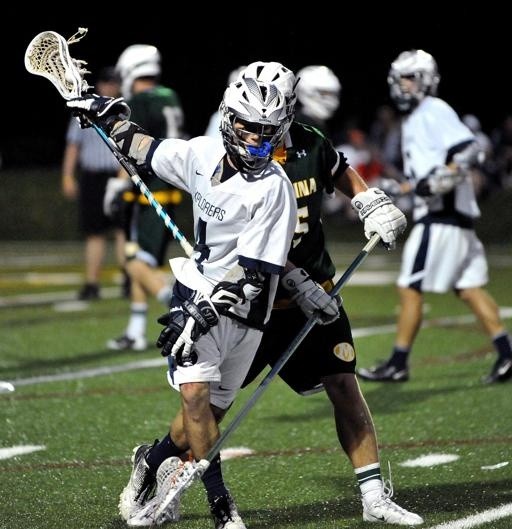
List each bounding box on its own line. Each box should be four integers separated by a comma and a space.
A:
356, 360, 409, 383
359, 492, 426, 526
107, 336, 150, 353
78, 284, 100, 300
480, 355, 512, 383
205, 487, 245, 529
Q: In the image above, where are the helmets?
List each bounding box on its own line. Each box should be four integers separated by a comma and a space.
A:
110, 44, 162, 100
215, 75, 290, 170
237, 60, 299, 124
387, 48, 442, 114
293, 64, 343, 120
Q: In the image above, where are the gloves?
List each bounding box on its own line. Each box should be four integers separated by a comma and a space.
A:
281, 267, 344, 326
154, 294, 220, 359
65, 93, 132, 129
351, 187, 408, 253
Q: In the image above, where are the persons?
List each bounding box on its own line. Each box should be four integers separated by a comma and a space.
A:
157, 60, 426, 527
68, 78, 296, 528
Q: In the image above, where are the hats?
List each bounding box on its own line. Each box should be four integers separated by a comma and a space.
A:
100, 66, 118, 82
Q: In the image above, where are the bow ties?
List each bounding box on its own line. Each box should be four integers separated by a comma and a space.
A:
117, 444, 180, 528
